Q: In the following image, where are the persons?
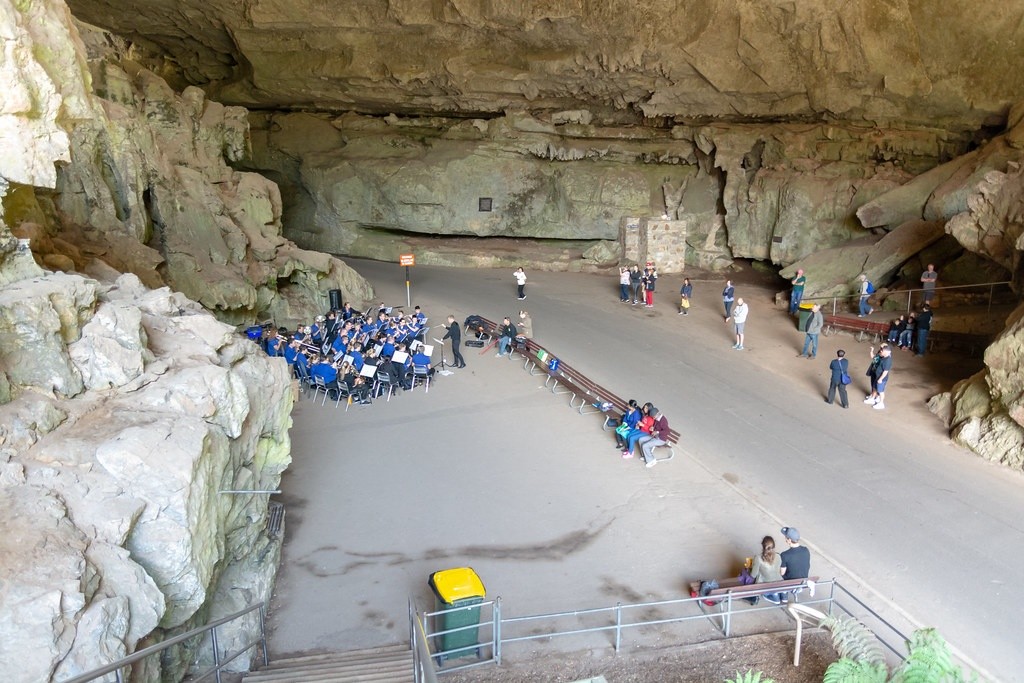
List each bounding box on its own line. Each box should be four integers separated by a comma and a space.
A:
744, 527, 810, 607
825, 349, 849, 409
789, 269, 806, 316
887, 304, 935, 358
641, 261, 658, 308
921, 264, 937, 304
857, 275, 874, 318
630, 264, 640, 306
619, 265, 631, 303
518, 310, 532, 357
723, 279, 734, 323
494, 316, 516, 357
796, 302, 824, 360
263, 302, 436, 404
678, 278, 692, 315
440, 315, 467, 369
615, 399, 670, 467
864, 343, 893, 410
732, 297, 749, 350
513, 266, 527, 301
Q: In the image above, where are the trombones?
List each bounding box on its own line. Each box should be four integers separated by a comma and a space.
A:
295, 341, 320, 352
276, 335, 288, 342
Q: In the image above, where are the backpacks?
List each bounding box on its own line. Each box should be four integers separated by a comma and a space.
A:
865, 281, 875, 294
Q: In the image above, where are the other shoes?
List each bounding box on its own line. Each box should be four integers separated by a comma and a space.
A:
646, 458, 657, 468
732, 343, 743, 350
763, 593, 781, 604
788, 310, 800, 317
517, 292, 732, 324
823, 391, 885, 409
616, 443, 634, 459
887, 336, 924, 357
796, 353, 815, 360
857, 309, 874, 318
780, 592, 789, 602
640, 457, 645, 460
317, 351, 516, 405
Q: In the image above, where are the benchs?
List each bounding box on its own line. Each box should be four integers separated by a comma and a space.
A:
691, 574, 820, 630
823, 315, 990, 361
463, 315, 681, 463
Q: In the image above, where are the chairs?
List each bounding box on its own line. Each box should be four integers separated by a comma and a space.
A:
375, 371, 400, 402
411, 363, 433, 393
312, 375, 338, 408
299, 360, 316, 399
335, 381, 363, 412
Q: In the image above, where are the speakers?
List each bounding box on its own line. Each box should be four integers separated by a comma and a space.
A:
330, 289, 342, 312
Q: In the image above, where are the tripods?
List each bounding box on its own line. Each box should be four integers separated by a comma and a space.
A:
433, 336, 450, 371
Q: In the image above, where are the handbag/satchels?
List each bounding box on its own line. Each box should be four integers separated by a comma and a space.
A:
842, 374, 852, 385
681, 295, 690, 308
866, 362, 876, 376
648, 279, 655, 291
737, 567, 755, 585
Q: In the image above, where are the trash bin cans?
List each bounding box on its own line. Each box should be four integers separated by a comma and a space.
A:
797, 302, 813, 332
430, 567, 485, 666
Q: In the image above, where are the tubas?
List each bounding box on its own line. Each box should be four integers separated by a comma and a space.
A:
314, 315, 327, 341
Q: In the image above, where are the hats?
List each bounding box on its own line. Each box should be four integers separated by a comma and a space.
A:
649, 408, 659, 417
781, 527, 800, 542
858, 275, 866, 280
645, 263, 651, 266
